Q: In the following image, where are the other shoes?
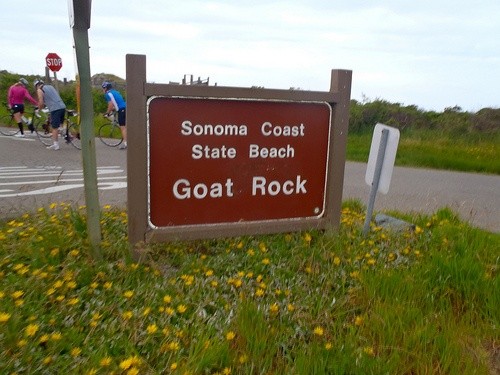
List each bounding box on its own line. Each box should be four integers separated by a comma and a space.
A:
65, 135, 74, 143
47, 144, 59, 150
16, 134, 25, 137
119, 141, 128, 149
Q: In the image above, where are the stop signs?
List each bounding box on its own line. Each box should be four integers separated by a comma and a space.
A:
46, 52, 62, 72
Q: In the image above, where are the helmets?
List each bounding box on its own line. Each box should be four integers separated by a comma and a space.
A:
102, 82, 112, 88
20, 78, 27, 84
33, 79, 43, 86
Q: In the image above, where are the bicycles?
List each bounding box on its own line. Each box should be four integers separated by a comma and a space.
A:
98, 109, 124, 148
33, 104, 83, 150
0, 101, 54, 138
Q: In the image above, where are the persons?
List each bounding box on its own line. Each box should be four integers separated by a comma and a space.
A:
33, 80, 73, 150
101, 82, 127, 150
8, 77, 39, 138
